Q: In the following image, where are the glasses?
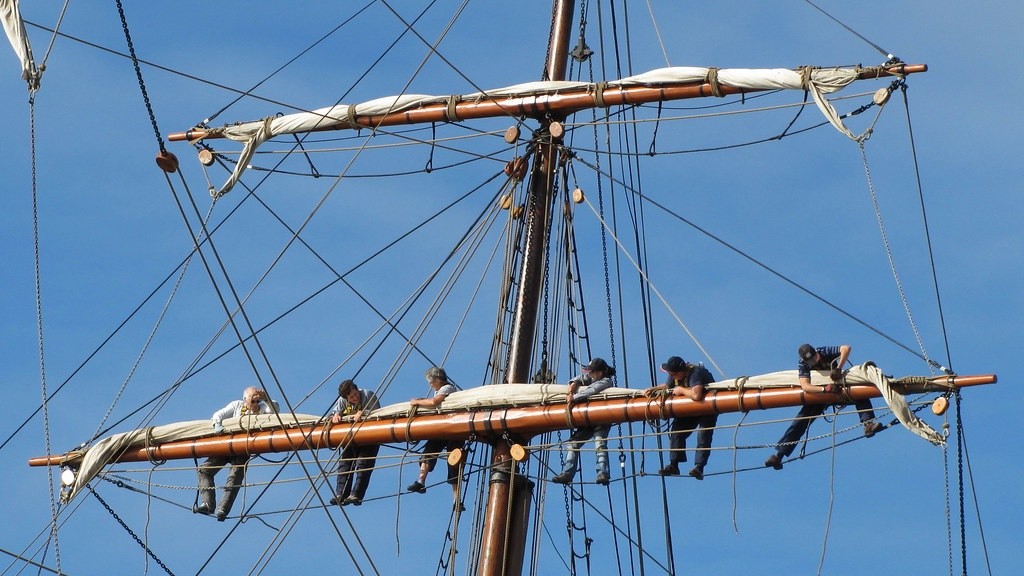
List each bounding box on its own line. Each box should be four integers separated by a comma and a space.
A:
250, 400, 262, 404
668, 372, 678, 378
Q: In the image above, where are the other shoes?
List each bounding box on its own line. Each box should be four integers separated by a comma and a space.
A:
552, 473, 573, 483
452, 499, 466, 512
865, 421, 882, 438
195, 501, 215, 515
330, 494, 345, 505
344, 494, 363, 506
596, 471, 609, 485
765, 456, 784, 470
658, 465, 680, 476
407, 481, 427, 494
689, 464, 703, 480
217, 508, 228, 522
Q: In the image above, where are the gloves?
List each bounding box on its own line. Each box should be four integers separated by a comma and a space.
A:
824, 383, 842, 395
252, 390, 267, 401
830, 368, 843, 381
214, 422, 223, 436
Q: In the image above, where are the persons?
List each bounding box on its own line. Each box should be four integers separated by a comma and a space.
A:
641, 356, 720, 481
330, 379, 381, 506
194, 386, 280, 522
763, 344, 883, 471
409, 366, 466, 512
551, 358, 616, 486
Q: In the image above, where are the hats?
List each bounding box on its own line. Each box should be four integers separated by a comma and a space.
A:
797, 343, 817, 362
582, 357, 606, 372
660, 355, 687, 373
